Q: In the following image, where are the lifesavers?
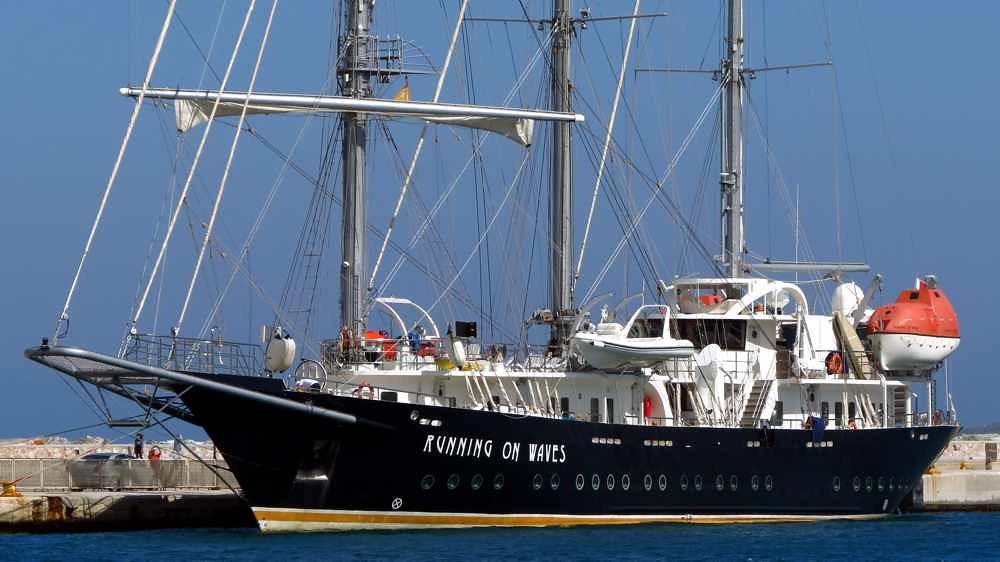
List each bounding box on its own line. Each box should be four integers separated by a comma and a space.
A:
831, 355, 842, 374
357, 381, 375, 400
849, 422, 857, 429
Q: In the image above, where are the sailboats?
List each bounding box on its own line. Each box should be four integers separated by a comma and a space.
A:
28, 1, 965, 519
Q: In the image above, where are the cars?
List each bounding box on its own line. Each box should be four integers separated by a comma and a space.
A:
72, 454, 137, 491
